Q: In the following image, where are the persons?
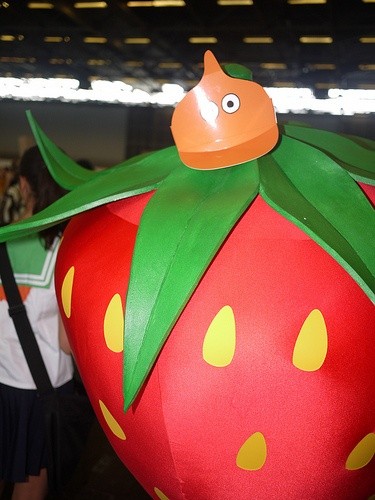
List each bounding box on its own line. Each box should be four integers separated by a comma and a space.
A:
1, 147, 74, 500
0, 147, 27, 228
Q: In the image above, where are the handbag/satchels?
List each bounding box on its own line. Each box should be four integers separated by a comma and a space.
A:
47, 376, 91, 499
